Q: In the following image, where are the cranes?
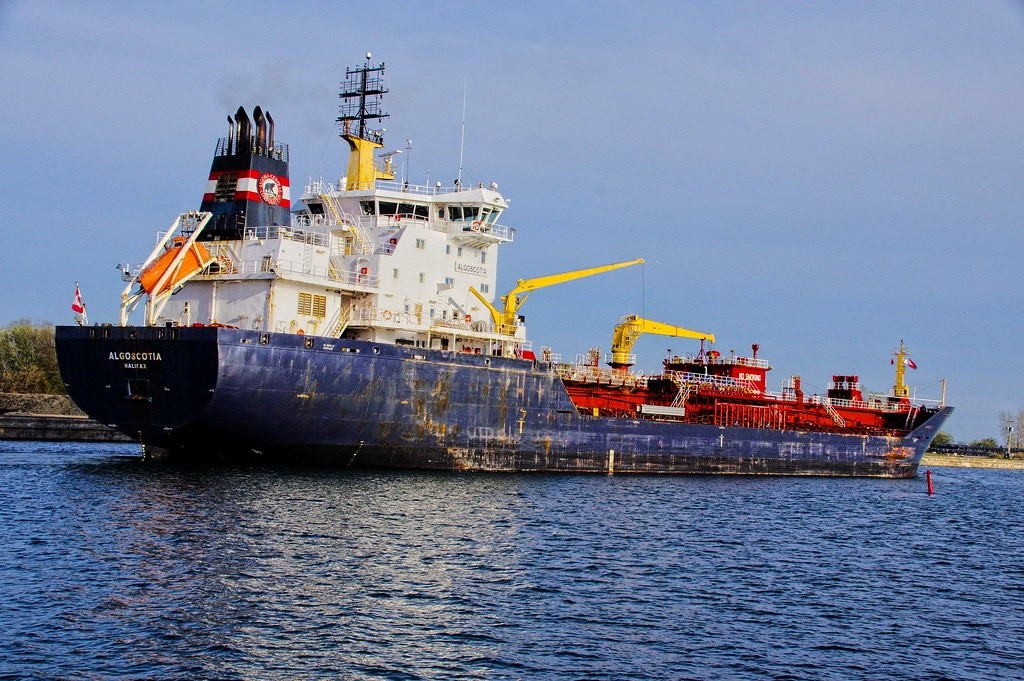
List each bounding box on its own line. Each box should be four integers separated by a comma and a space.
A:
608, 314, 716, 370
469, 257, 645, 337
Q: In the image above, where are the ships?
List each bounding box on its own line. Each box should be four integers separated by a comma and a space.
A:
54, 50, 957, 476
